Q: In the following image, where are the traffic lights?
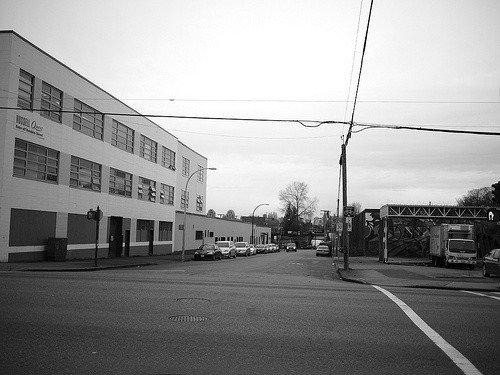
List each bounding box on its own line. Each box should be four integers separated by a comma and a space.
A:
87, 211, 96, 219
487, 208, 497, 221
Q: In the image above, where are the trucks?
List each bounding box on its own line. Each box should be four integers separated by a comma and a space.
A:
430, 223, 480, 270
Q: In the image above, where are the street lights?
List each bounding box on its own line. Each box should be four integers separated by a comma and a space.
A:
252, 204, 269, 244
182, 168, 217, 256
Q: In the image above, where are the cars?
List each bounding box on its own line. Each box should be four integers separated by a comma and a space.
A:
215, 241, 237, 259
287, 242, 296, 252
249, 243, 280, 255
482, 249, 500, 277
316, 245, 331, 256
234, 241, 250, 256
194, 244, 222, 260
